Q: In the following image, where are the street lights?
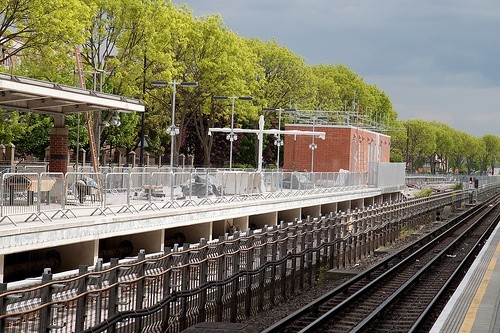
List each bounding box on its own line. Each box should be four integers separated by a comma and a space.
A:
151, 81, 198, 203
262, 107, 297, 195
301, 116, 328, 190
213, 95, 253, 200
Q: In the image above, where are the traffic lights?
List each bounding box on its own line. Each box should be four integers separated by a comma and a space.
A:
469, 177, 472, 184
475, 180, 478, 188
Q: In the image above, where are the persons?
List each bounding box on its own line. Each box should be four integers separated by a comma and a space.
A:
195, 176, 222, 196
83, 174, 101, 189
